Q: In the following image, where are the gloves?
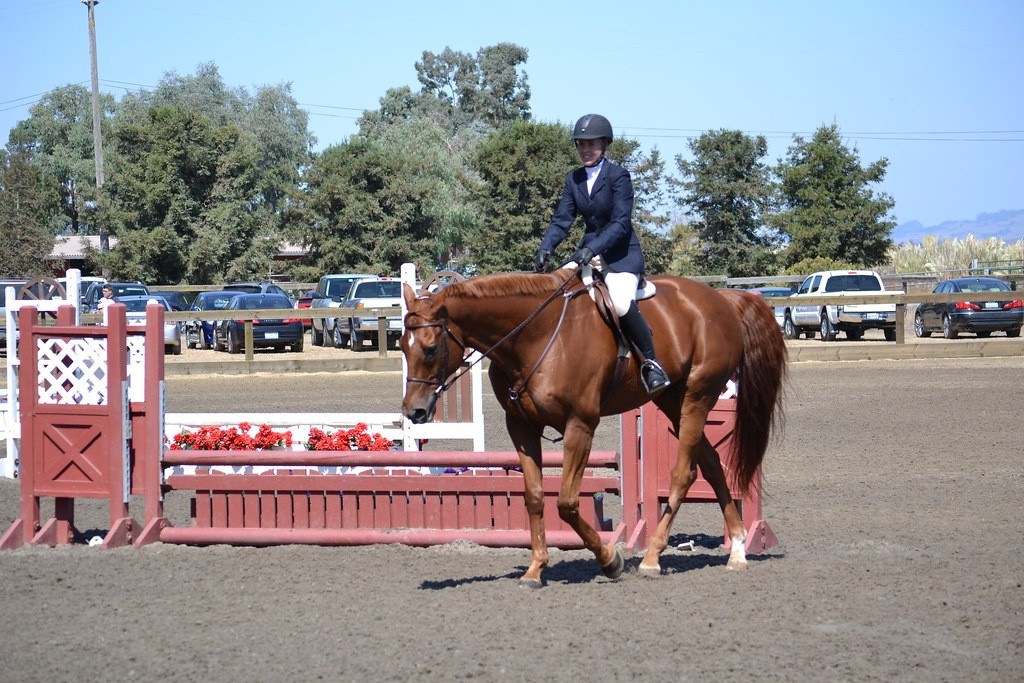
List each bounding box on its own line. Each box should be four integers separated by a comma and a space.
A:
571, 245, 595, 266
533, 250, 551, 268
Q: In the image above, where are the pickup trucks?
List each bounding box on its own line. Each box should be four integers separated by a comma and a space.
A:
782, 270, 907, 341
330, 277, 404, 352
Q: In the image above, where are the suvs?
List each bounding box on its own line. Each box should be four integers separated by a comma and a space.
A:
310, 270, 383, 346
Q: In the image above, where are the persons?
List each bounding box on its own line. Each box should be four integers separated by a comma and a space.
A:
102, 284, 120, 303
536, 114, 667, 388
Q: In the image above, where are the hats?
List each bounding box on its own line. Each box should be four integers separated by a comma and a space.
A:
570, 113, 613, 140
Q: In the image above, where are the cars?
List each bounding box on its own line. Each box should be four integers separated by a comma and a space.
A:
750, 283, 792, 329
184, 291, 251, 350
913, 277, 1024, 340
0, 274, 316, 357
211, 292, 304, 353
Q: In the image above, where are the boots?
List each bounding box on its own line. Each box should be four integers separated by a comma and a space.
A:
617, 298, 669, 389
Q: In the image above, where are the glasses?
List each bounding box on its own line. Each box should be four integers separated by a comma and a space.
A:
102, 290, 109, 292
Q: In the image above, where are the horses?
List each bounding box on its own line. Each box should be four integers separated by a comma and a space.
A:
398, 267, 790, 590
